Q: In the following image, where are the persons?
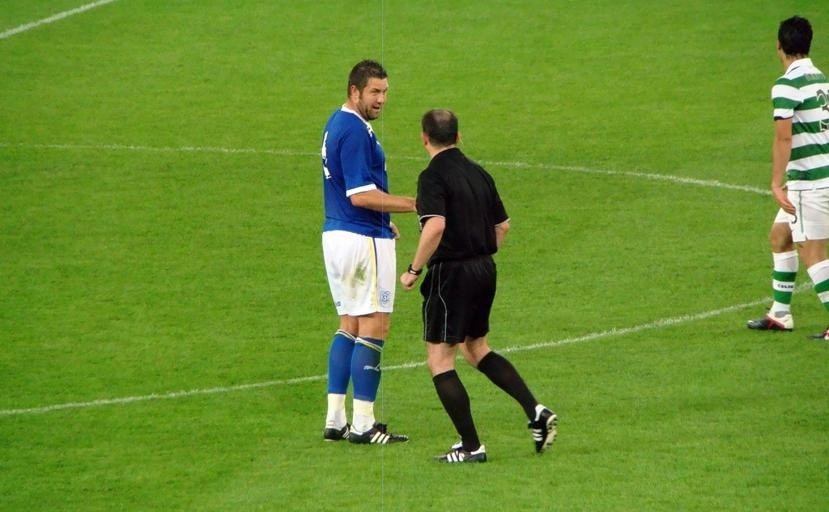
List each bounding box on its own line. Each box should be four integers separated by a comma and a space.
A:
743, 14, 828, 341
319, 60, 416, 445
397, 107, 559, 466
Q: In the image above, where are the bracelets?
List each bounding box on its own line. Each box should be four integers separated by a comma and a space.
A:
407, 263, 422, 275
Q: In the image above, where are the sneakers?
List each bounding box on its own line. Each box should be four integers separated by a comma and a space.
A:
526, 403, 557, 455
806, 327, 829, 341
745, 312, 793, 331
349, 422, 410, 445
432, 440, 486, 464
323, 420, 352, 441
